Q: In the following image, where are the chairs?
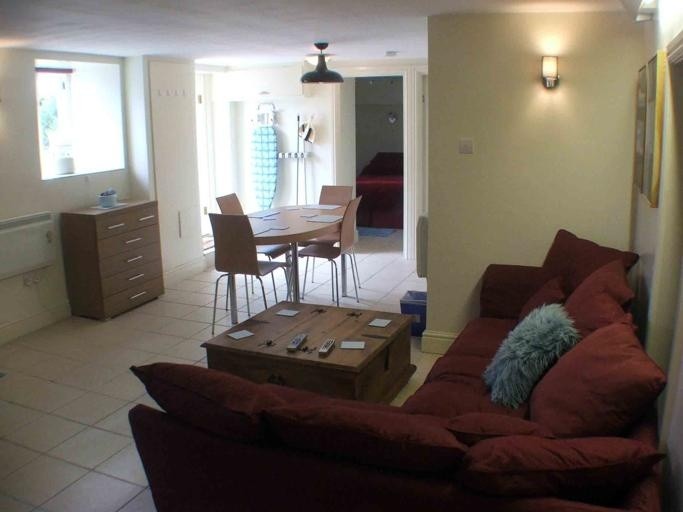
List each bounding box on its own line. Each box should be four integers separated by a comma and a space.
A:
206, 178, 362, 338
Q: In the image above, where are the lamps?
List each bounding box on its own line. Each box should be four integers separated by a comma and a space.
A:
539, 54, 559, 89
299, 40, 343, 85
291, 112, 317, 206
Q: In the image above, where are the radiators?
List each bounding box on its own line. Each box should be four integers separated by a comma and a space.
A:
0, 210, 56, 281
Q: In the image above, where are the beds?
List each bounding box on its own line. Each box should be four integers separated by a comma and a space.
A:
356, 151, 404, 228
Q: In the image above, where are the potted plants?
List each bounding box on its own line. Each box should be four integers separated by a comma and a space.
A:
96, 186, 118, 207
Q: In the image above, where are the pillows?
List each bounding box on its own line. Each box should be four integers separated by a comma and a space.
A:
482, 303, 580, 407
447, 412, 553, 446
572, 296, 637, 346
289, 402, 459, 450
517, 279, 563, 324
128, 360, 293, 420
530, 312, 662, 440
563, 263, 630, 314
535, 228, 640, 289
465, 435, 665, 478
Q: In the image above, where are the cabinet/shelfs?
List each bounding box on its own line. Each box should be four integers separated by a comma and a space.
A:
58, 199, 166, 321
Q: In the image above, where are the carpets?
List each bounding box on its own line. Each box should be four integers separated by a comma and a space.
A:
356, 227, 396, 238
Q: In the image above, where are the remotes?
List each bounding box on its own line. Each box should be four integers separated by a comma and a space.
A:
287, 334, 307, 351
318, 339, 335, 353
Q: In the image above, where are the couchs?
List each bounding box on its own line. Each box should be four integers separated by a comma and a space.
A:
125, 262, 659, 509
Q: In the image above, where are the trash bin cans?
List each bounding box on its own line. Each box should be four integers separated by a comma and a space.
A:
400, 290, 426, 336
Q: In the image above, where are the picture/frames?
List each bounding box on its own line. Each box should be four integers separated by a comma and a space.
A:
632, 59, 648, 195
641, 44, 666, 209
